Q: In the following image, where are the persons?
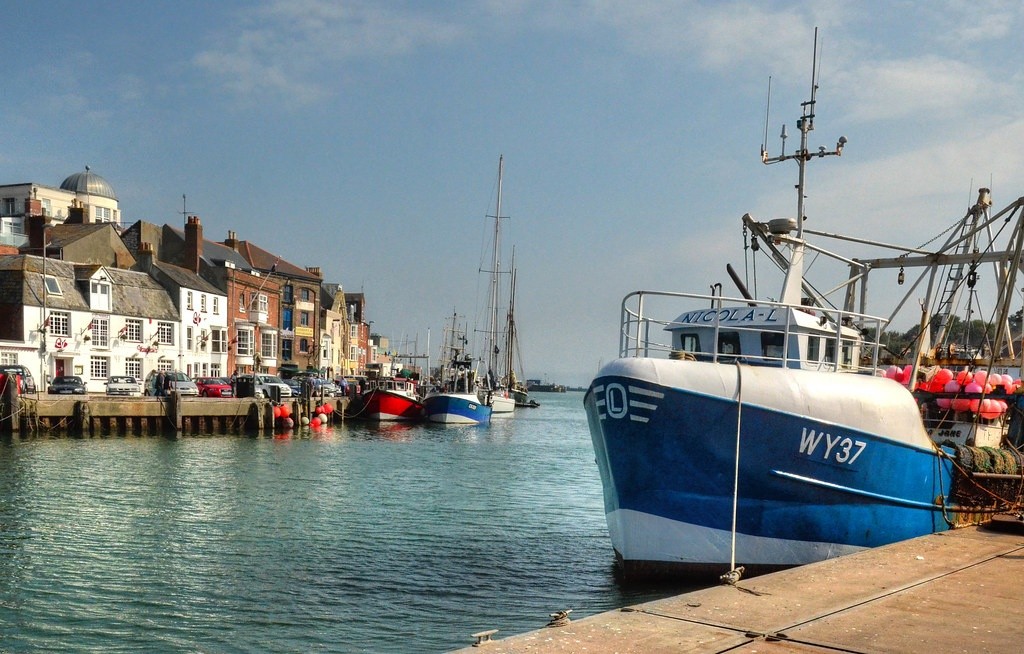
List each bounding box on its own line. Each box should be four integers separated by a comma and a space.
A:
144, 371, 172, 397
338, 375, 347, 396
359, 378, 366, 395
436, 378, 441, 390
306, 373, 321, 398
230, 369, 239, 397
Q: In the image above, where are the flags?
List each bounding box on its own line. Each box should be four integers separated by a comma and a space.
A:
44, 316, 49, 326
88, 322, 93, 330
231, 337, 237, 343
204, 336, 208, 341
158, 327, 160, 334
121, 327, 127, 333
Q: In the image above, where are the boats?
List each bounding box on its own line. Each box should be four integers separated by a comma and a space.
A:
583, 27, 1024, 594
362, 153, 540, 424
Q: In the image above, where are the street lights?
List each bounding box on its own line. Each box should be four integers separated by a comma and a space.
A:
42, 223, 55, 393
366, 320, 375, 364
179, 282, 186, 372
231, 268, 242, 375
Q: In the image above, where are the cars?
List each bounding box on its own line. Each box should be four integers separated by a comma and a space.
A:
47, 376, 88, 395
144, 369, 199, 397
0, 365, 36, 394
190, 373, 368, 398
103, 376, 142, 397
